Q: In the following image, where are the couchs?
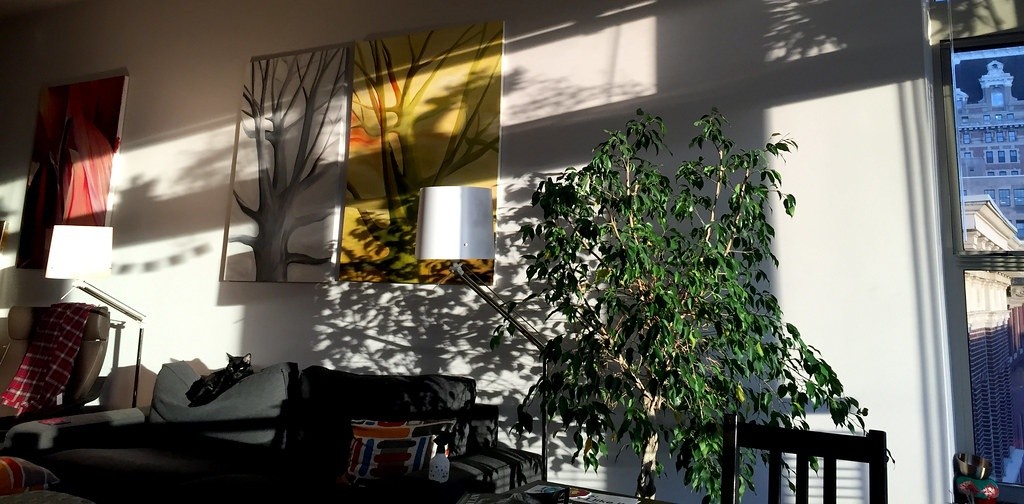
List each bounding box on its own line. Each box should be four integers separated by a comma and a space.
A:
1, 360, 544, 504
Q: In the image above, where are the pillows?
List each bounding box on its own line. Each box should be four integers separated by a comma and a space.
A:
0, 455, 60, 494
346, 416, 457, 497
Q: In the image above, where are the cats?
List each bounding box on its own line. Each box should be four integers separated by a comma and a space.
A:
184, 353, 254, 408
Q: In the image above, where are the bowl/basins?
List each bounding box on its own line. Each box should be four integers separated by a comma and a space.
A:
956, 453, 993, 479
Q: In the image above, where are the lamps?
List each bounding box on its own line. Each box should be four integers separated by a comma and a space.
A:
45, 224, 146, 408
415, 186, 555, 482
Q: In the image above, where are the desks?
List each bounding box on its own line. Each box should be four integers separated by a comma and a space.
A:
498, 479, 672, 504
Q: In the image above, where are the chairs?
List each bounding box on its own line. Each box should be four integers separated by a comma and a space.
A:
724, 413, 890, 504
0, 304, 110, 440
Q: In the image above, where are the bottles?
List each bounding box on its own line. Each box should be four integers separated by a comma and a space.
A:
428, 433, 454, 483
